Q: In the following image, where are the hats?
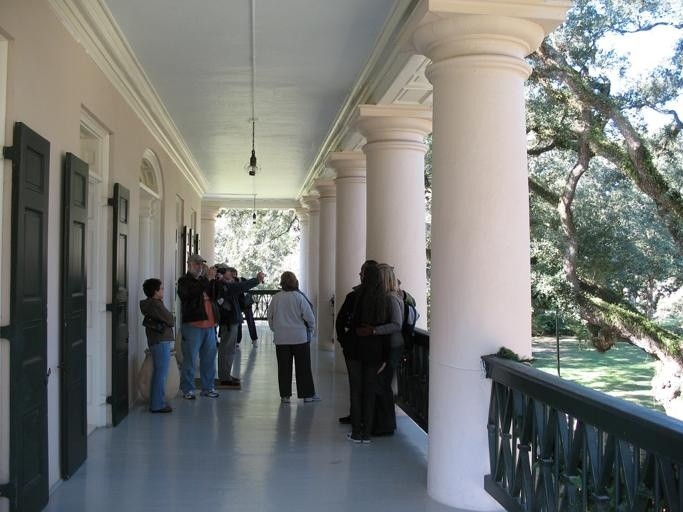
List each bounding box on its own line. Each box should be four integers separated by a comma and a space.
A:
188, 254, 207, 263
217, 263, 233, 270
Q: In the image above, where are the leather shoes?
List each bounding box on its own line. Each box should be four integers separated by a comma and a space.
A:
152, 406, 172, 413
232, 377, 238, 381
220, 380, 240, 386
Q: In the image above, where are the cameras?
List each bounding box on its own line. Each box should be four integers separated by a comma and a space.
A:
214, 265, 226, 275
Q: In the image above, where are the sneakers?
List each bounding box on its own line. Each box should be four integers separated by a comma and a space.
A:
304, 396, 322, 402
201, 388, 219, 397
340, 413, 394, 445
185, 391, 196, 400
252, 338, 258, 348
280, 397, 291, 403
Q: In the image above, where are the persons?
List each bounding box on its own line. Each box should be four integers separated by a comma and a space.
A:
138, 279, 176, 409
177, 255, 263, 399
266, 272, 322, 403
335, 260, 419, 443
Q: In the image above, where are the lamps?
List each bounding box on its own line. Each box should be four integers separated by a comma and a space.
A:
253, 194, 256, 224
244, 119, 258, 175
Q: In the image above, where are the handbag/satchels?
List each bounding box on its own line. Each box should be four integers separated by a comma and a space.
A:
142, 314, 165, 334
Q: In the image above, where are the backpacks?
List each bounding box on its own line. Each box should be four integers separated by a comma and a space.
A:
403, 303, 417, 350
